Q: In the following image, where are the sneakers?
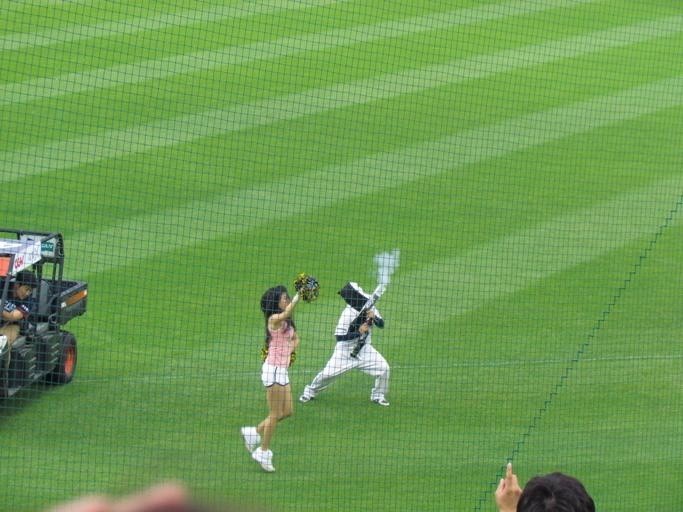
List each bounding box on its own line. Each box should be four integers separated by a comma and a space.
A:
241, 426, 262, 454
251, 446, 276, 472
370, 395, 390, 406
299, 394, 311, 404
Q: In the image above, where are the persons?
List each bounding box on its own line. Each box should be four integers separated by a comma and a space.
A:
300, 280, 390, 409
240, 284, 310, 472
493, 462, 595, 512
0, 269, 36, 350
51, 480, 187, 512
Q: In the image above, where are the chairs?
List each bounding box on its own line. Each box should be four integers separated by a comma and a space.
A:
27, 279, 53, 336
